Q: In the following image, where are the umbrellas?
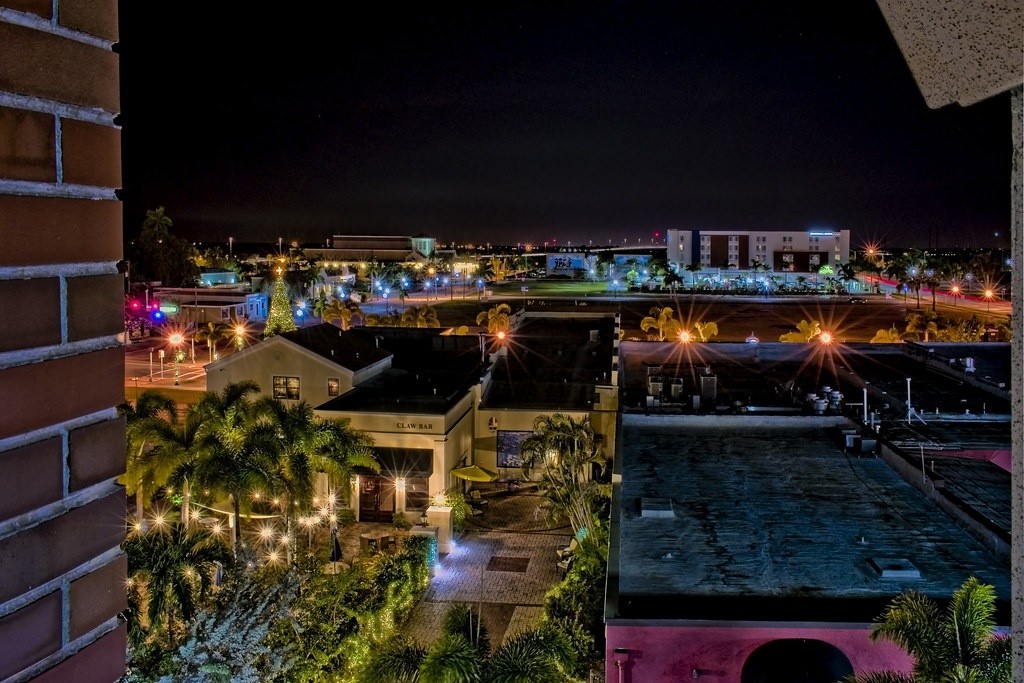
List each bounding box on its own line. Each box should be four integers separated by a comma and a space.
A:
451, 465, 499, 506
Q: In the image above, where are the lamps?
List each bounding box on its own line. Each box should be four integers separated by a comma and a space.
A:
420, 513, 427, 527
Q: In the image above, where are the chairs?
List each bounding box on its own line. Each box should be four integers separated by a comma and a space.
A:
359, 536, 368, 558
470, 490, 489, 512
344, 554, 354, 563
389, 531, 396, 549
377, 536, 390, 553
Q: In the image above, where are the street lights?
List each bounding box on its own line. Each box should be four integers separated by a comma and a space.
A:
401, 277, 410, 310
613, 279, 619, 298
450, 273, 460, 300
953, 286, 958, 306
383, 288, 391, 311
986, 290, 991, 312
299, 300, 306, 326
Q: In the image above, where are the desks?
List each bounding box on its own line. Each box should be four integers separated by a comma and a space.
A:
319, 562, 351, 575
464, 498, 483, 509
360, 530, 389, 550
